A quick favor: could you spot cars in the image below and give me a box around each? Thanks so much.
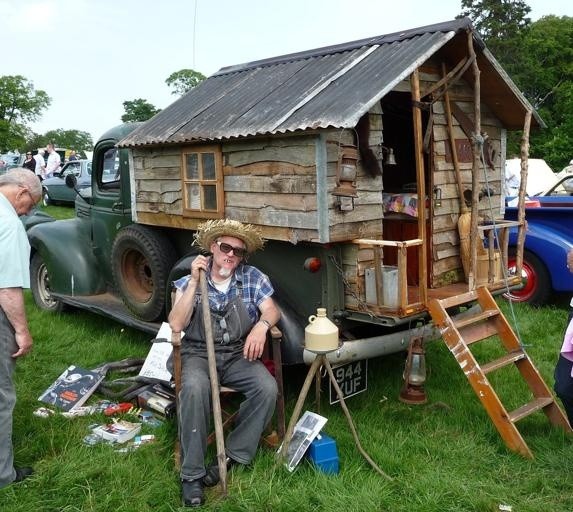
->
[481,156,572,305]
[1,146,121,207]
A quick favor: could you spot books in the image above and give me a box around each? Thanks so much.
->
[32,365,170,454]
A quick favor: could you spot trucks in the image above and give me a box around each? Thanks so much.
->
[22,121,475,406]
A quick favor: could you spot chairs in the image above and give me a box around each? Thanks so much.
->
[170,286,284,469]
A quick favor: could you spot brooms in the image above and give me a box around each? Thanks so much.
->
[442,63,486,282]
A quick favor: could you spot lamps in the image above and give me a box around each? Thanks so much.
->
[397,317,427,404]
[331,128,359,211]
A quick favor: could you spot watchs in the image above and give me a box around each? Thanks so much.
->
[258,319,274,329]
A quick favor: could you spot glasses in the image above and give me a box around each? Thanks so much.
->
[26,154,31,155]
[214,239,246,257]
[18,185,40,210]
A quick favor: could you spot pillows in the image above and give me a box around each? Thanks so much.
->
[383,193,429,217]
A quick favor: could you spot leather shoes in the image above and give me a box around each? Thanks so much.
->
[180,478,204,507]
[12,466,33,483]
[202,450,236,487]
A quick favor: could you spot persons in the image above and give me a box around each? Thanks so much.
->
[554,247,573,431]
[0,143,63,181]
[69,149,77,161]
[167,219,284,508]
[0,166,44,488]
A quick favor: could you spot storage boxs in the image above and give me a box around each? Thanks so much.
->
[307,431,339,475]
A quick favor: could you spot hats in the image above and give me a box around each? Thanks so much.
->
[189,218,265,256]
